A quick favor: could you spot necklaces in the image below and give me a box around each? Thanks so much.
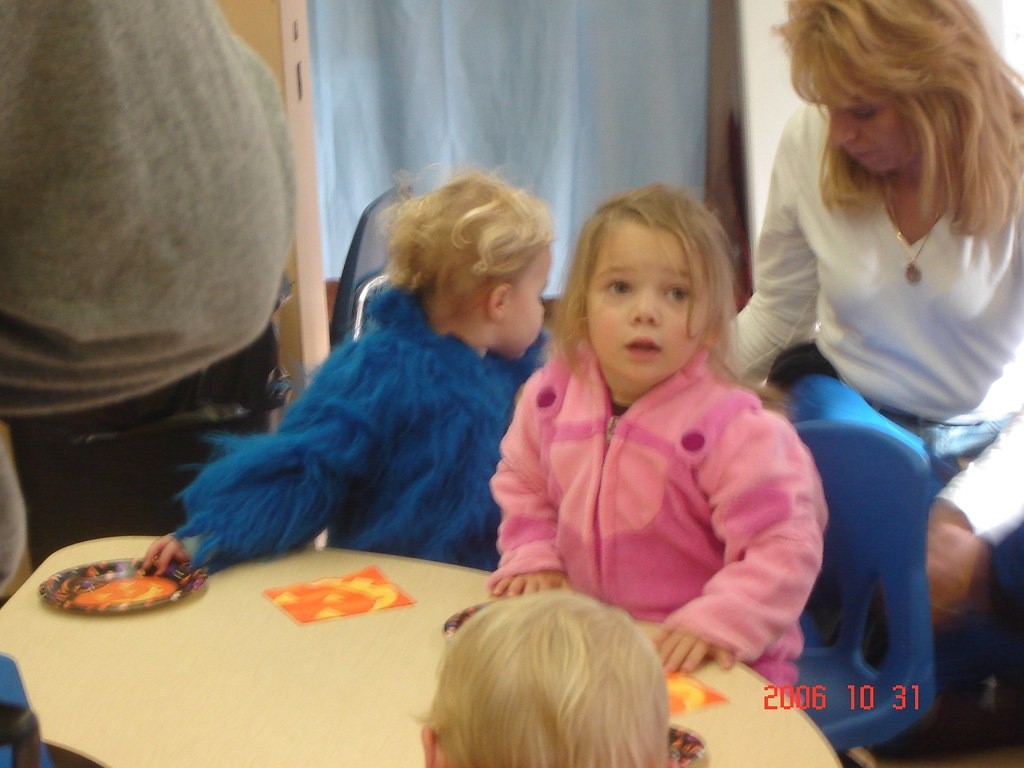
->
[870,174,945,286]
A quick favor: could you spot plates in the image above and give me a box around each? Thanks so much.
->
[38,558,207,612]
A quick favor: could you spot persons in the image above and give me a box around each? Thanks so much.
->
[138,170,555,584]
[712,9,1023,638]
[2,1,298,568]
[485,187,829,689]
[423,591,674,768]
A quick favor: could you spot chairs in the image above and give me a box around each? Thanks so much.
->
[330,186,411,348]
[785,372,937,768]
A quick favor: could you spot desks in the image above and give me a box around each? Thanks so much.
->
[0,534,844,768]
